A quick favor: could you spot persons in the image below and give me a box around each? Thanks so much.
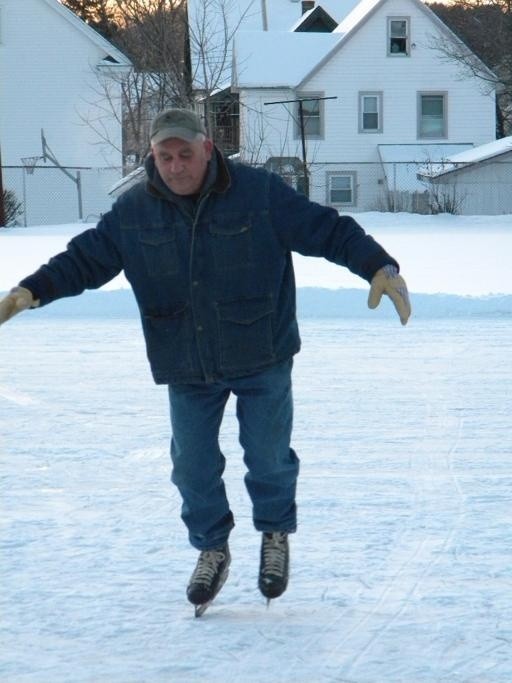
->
[0,108,414,606]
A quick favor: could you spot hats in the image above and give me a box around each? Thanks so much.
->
[148,107,206,147]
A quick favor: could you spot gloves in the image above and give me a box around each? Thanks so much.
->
[366,261,411,328]
[0,285,40,326]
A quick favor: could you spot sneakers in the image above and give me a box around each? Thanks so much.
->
[186,540,232,606]
[257,528,291,599]
